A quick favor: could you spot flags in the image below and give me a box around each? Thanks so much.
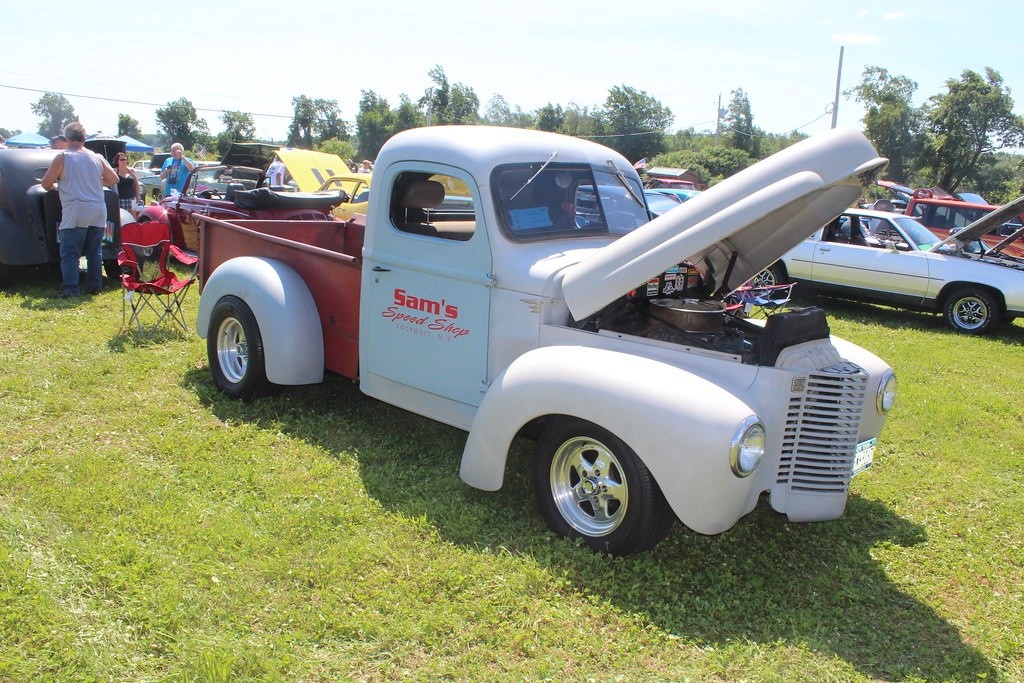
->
[634,158,645,169]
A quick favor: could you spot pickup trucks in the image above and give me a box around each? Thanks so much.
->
[192,118,900,557]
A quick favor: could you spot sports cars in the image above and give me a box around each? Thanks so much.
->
[135,142,348,263]
[840,214,1024,261]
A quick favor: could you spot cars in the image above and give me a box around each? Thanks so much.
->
[270,147,373,223]
[128,147,221,196]
[0,147,146,284]
[570,177,706,228]
[746,196,1024,335]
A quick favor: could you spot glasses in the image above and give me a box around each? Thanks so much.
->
[119,159,127,161]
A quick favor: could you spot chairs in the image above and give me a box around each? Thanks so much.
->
[397,180,445,235]
[735,278,798,318]
[114,222,200,338]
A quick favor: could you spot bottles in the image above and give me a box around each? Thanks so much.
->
[169,188,181,197]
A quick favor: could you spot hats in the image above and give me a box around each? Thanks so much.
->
[51,135,66,142]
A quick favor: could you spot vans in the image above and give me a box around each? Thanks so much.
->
[902,197,1023,258]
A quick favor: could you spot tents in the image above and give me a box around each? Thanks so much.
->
[118,136,155,156]
[85,130,127,167]
[5,132,52,147]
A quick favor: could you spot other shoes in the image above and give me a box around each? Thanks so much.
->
[61,290,80,298]
[86,286,101,295]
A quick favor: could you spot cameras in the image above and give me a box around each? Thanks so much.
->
[167,174,176,184]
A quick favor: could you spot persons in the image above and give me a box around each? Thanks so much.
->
[159,143,195,197]
[42,123,119,297]
[0,135,8,149]
[114,153,139,219]
[347,159,371,173]
[266,155,292,186]
[643,178,649,189]
